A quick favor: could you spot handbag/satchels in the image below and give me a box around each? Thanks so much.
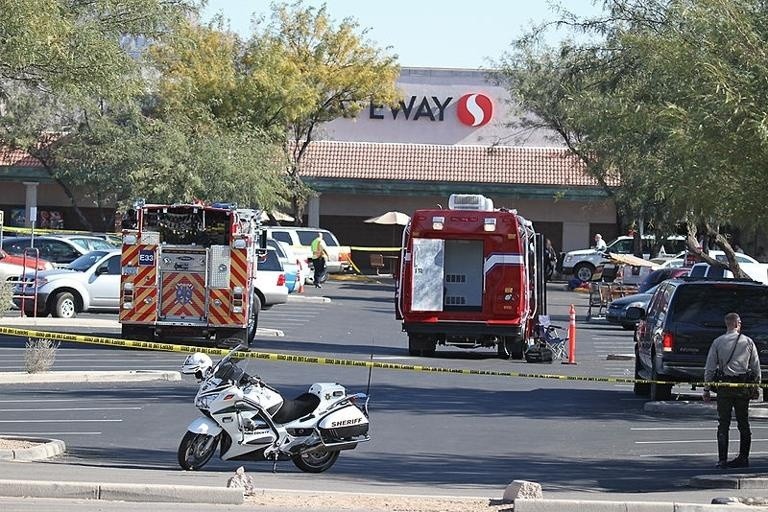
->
[710,368,723,392]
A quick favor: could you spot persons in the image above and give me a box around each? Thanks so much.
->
[595,234,606,250]
[544,238,558,282]
[703,313,762,468]
[311,233,330,288]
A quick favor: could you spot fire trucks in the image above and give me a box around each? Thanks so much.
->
[393,191,556,362]
[117,197,271,350]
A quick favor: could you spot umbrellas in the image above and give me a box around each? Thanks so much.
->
[363,211,412,273]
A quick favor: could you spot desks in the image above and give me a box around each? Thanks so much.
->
[383,256,399,273]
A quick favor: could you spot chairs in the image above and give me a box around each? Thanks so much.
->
[369,254,385,274]
[586,282,637,322]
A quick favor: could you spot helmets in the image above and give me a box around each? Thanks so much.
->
[181,352,212,380]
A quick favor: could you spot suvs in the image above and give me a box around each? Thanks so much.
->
[633,278,768,402]
[562,233,691,284]
[13,249,122,318]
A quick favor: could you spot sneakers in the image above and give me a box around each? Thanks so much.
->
[716,457,748,468]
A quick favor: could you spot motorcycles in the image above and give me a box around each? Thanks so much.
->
[173,339,377,474]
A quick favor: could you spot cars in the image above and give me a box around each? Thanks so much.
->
[602,248,768,328]
[0,223,122,308]
[253,220,351,314]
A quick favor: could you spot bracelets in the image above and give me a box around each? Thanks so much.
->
[703,388,710,392]
